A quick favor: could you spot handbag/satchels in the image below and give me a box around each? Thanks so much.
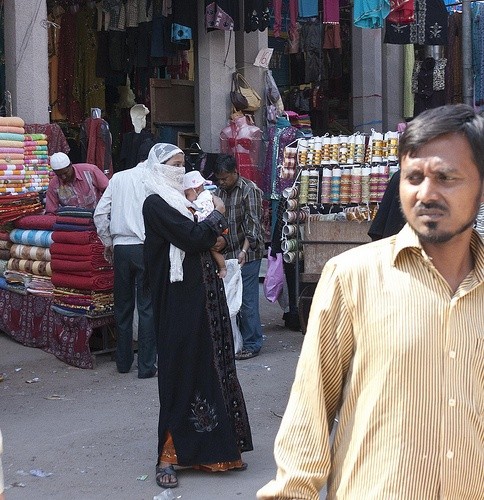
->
[263,246,285,303]
[230,72,261,111]
[264,71,279,103]
[277,265,290,313]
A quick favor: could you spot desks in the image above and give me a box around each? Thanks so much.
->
[0,277,139,369]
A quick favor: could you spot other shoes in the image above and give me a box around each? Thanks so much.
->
[235,350,258,360]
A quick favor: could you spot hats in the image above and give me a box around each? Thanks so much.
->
[50,152,71,170]
[183,170,212,191]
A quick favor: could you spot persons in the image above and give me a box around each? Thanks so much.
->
[92,138,158,379]
[183,169,228,278]
[257,103,484,500]
[260,116,305,202]
[210,153,265,359]
[117,104,155,173]
[221,115,264,188]
[83,109,113,181]
[141,143,254,488]
[44,152,110,214]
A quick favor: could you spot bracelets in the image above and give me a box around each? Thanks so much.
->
[242,249,246,253]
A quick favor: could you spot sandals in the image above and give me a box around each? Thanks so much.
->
[229,462,248,471]
[156,464,178,488]
